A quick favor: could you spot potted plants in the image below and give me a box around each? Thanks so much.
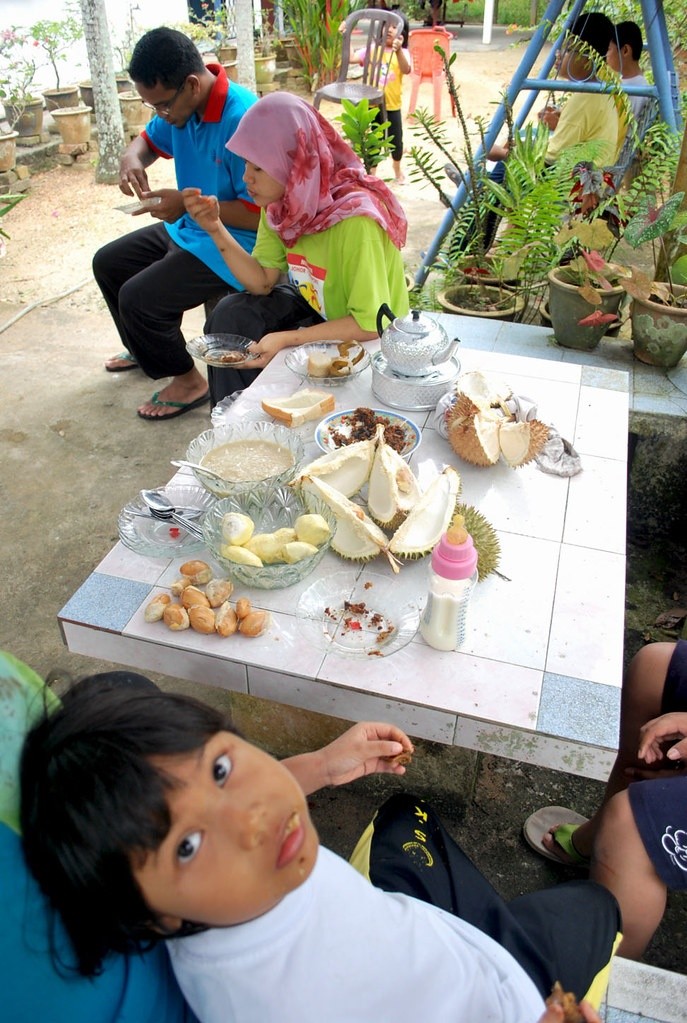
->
[408,19,687,367]
[0,0,286,173]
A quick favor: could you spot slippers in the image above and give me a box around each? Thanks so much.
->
[138,389,210,420]
[106,351,139,372]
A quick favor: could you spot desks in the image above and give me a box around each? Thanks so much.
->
[58,326,629,781]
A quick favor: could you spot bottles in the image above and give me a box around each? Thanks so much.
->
[418,513,479,652]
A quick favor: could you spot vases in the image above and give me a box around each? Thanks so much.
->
[280,37,303,68]
[78,80,95,114]
[50,105,93,143]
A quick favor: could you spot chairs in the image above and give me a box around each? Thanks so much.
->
[314,9,404,153]
[407,26,456,126]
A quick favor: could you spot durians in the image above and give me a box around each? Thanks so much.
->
[220,510,329,567]
[447,392,551,465]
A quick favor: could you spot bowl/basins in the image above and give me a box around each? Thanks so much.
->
[284,339,372,386]
[201,486,337,591]
[314,409,422,459]
[186,420,305,499]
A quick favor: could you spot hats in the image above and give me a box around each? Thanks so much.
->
[570,12,615,62]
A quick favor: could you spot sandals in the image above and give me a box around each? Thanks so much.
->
[521,806,596,871]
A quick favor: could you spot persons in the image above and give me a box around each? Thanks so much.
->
[18,687,624,1023]
[523,639,687,958]
[338,10,413,185]
[445,21,649,189]
[181,91,410,414]
[91,26,259,420]
[419,13,630,269]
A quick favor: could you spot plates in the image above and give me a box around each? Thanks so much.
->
[120,540,206,558]
[117,483,219,550]
[297,569,422,662]
[117,534,206,554]
[186,333,259,368]
[208,382,345,448]
[112,195,162,214]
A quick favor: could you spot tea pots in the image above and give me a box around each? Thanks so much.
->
[376,302,461,377]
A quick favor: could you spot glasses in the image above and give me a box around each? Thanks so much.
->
[141,72,197,116]
[555,47,591,58]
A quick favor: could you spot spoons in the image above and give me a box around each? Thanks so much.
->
[138,488,204,542]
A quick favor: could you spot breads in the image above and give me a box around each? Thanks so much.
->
[261,387,334,426]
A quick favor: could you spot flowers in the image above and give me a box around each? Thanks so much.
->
[0,25,50,101]
